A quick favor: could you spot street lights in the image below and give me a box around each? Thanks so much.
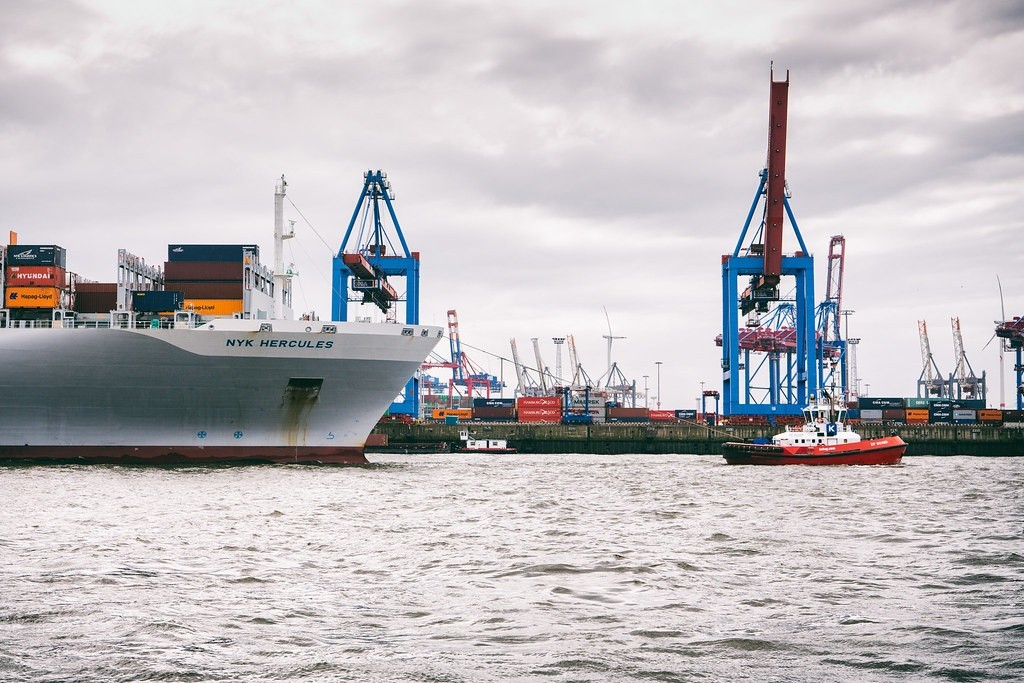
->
[643,375,649,407]
[696,397,701,413]
[700,381,705,391]
[655,362,663,410]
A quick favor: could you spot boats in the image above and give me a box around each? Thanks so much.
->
[460,439,517,454]
[722,385,910,466]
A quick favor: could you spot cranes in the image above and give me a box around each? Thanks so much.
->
[918,315,988,402]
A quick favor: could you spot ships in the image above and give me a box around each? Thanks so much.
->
[0,244,444,471]
